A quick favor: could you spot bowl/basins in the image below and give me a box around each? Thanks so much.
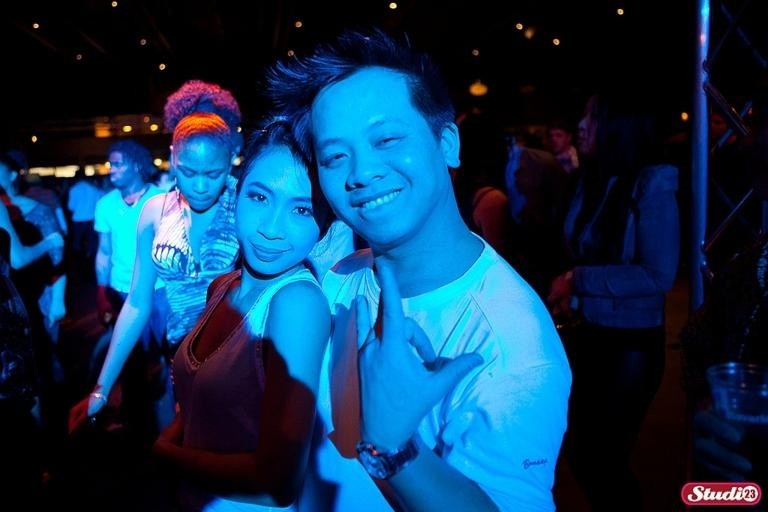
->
[709,360,768,427]
